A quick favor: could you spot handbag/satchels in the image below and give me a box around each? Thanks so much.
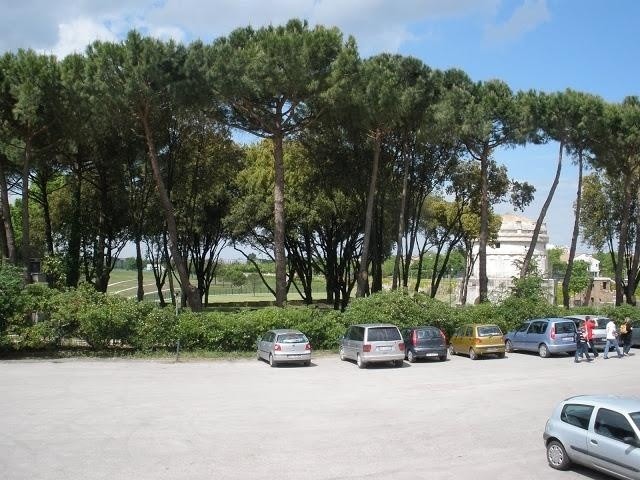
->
[620,325,628,334]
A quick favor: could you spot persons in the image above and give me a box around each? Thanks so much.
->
[603,317,624,359]
[575,316,600,363]
[622,316,632,355]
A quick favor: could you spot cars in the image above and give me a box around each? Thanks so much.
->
[617,320,640,348]
[564,314,616,350]
[448,323,506,360]
[504,318,578,358]
[542,394,640,480]
[258,328,311,366]
[400,326,449,361]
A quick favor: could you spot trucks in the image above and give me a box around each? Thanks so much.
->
[146,264,166,271]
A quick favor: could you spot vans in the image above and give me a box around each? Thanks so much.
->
[337,323,406,368]
